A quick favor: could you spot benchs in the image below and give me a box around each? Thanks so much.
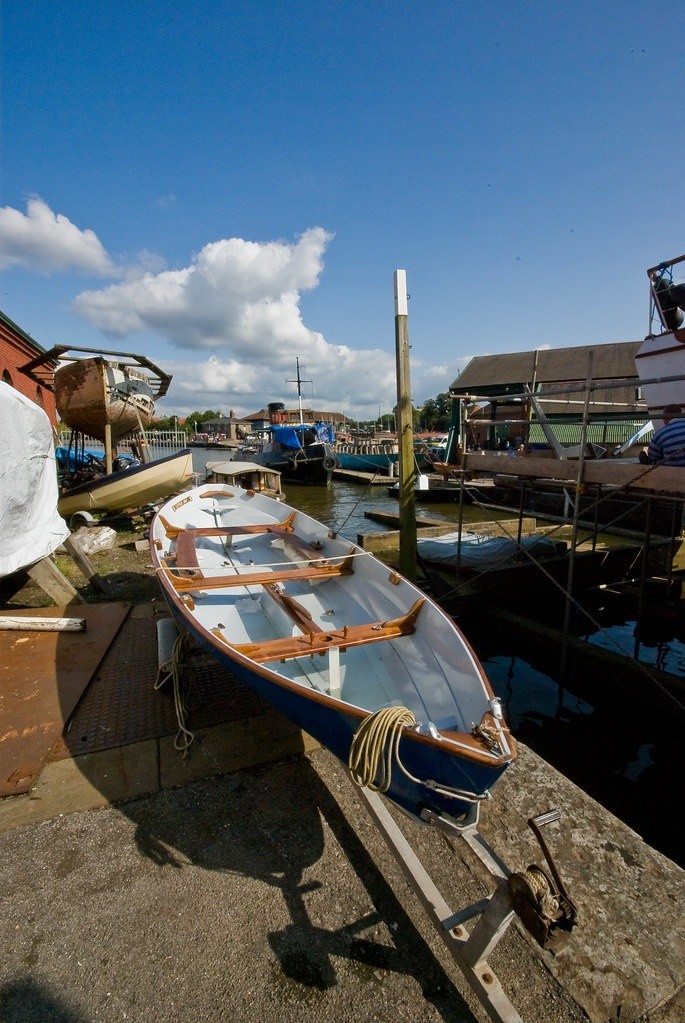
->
[162,545,354,593]
[177,529,203,579]
[268,528,335,567]
[212,597,425,662]
[159,510,296,538]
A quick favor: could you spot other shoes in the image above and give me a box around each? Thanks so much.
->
[639,451,648,465]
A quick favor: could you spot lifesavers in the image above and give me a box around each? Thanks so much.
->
[287,460,297,472]
[322,456,337,472]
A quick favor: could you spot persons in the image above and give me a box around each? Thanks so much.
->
[639,404,685,466]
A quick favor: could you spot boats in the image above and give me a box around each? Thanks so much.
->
[57,447,194,519]
[334,438,446,473]
[230,357,338,485]
[201,461,287,504]
[386,482,481,502]
[150,481,519,835]
[52,356,157,445]
